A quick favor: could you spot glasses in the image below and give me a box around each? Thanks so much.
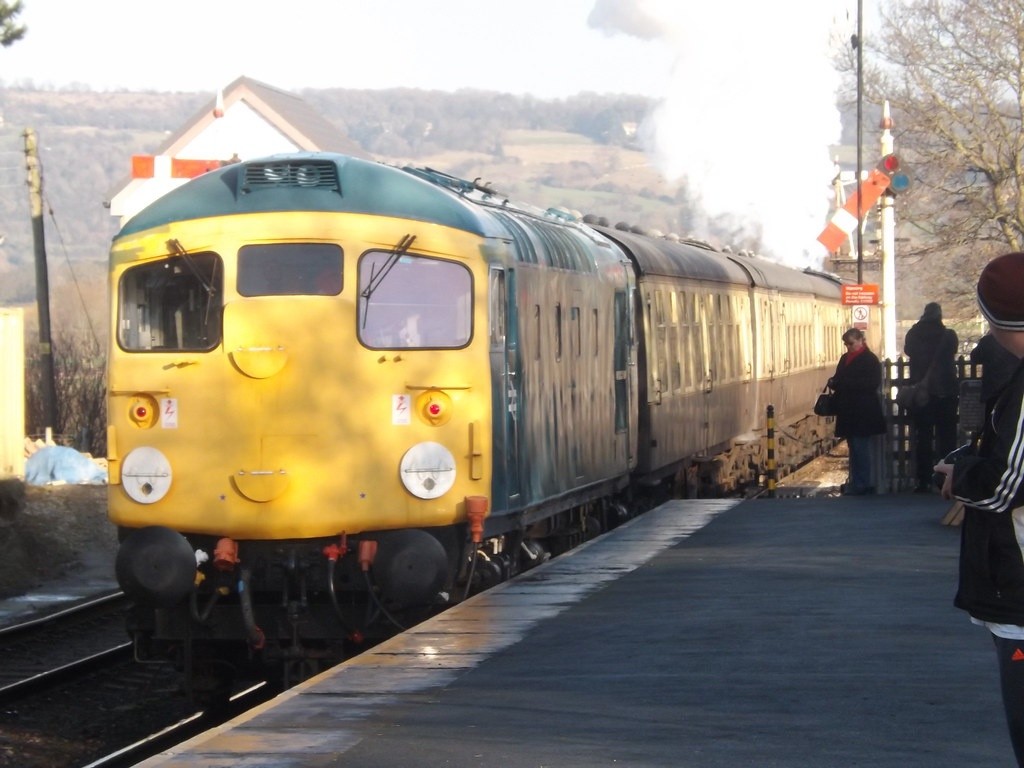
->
[844,342,854,345]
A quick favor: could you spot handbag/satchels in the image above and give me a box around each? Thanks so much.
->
[814,385,837,416]
[896,384,928,410]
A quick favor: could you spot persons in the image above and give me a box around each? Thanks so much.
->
[970,329,1020,423]
[904,302,958,492]
[826,328,882,497]
[932,255,1024,767]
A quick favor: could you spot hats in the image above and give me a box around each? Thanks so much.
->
[977,253,1024,332]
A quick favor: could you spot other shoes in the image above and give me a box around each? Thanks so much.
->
[848,489,877,505]
[913,484,931,496]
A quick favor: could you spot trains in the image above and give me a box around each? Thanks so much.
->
[95,152,884,670]
